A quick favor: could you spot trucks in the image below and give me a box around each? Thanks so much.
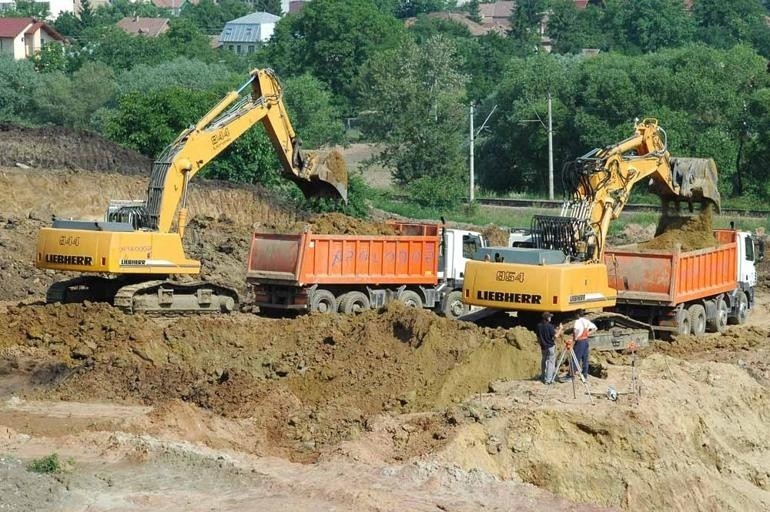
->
[600,220,765,344]
[247,214,488,323]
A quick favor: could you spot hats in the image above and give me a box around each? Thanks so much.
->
[543,312,553,318]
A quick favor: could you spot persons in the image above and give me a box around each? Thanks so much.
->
[536,311,563,385]
[561,309,599,383]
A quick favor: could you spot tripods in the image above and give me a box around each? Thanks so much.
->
[542,345,595,405]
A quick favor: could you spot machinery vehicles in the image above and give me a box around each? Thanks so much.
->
[459,116,720,353]
[39,64,350,313]
